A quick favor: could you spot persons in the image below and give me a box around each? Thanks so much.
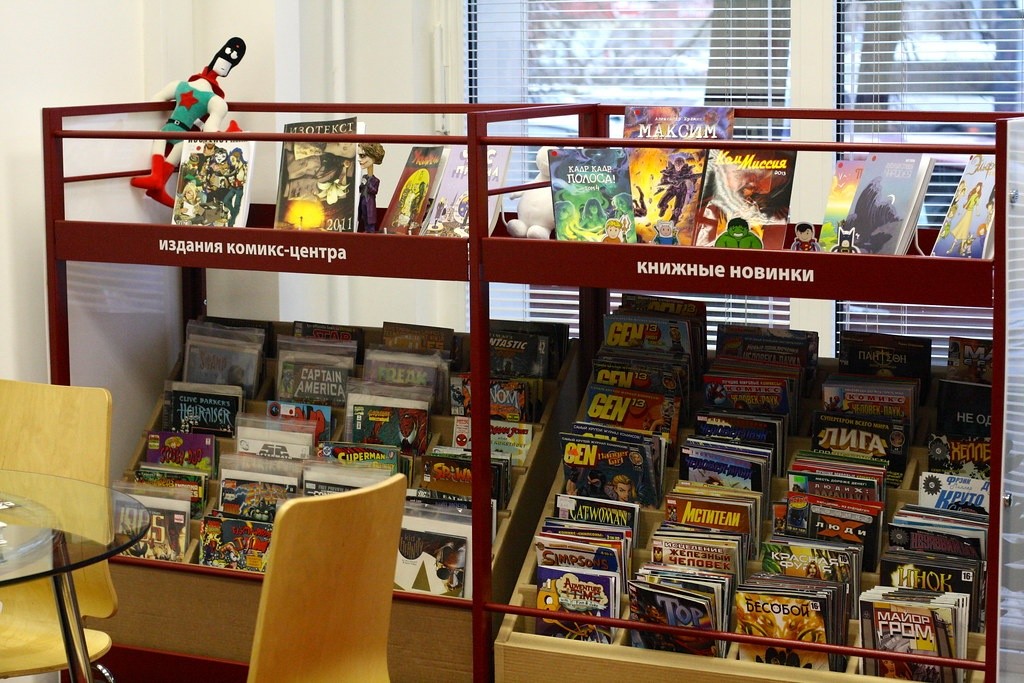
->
[356,143,385,233]
[130,36,246,208]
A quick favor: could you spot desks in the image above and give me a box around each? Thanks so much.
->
[0,468,150,683]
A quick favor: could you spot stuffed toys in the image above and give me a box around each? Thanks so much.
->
[501,146,563,240]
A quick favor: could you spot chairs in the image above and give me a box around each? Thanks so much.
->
[248,475,407,683]
[0,377,118,683]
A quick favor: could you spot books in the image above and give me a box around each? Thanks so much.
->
[273,116,366,235]
[548,106,800,249]
[819,152,936,255]
[379,145,515,239]
[172,140,256,229]
[931,155,996,259]
[110,291,993,683]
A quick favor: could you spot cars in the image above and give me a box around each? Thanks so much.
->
[526,119,623,182]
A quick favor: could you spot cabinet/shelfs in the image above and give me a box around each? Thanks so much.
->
[43,101,1024,681]
